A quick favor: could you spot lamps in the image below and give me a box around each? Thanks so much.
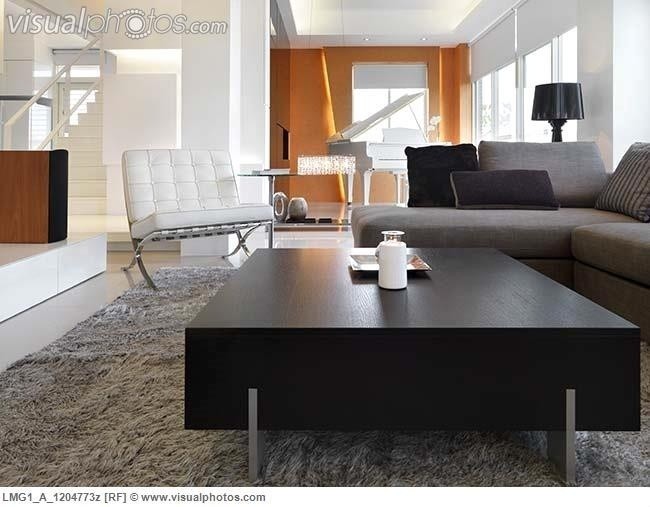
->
[531,83,584,142]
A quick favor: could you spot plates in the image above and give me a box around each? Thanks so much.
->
[348,254,433,274]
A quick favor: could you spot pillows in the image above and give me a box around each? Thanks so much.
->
[450,169,561,209]
[405,144,478,208]
[594,142,650,222]
[478,141,608,208]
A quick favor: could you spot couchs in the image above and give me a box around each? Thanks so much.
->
[351,203,643,292]
[571,222,650,349]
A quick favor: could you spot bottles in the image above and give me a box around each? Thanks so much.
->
[377,230,408,291]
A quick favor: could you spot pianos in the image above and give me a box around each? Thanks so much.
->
[325,93,453,208]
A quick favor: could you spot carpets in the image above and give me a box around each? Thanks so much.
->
[0,267,650,487]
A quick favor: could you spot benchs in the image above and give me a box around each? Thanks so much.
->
[121,150,274,289]
